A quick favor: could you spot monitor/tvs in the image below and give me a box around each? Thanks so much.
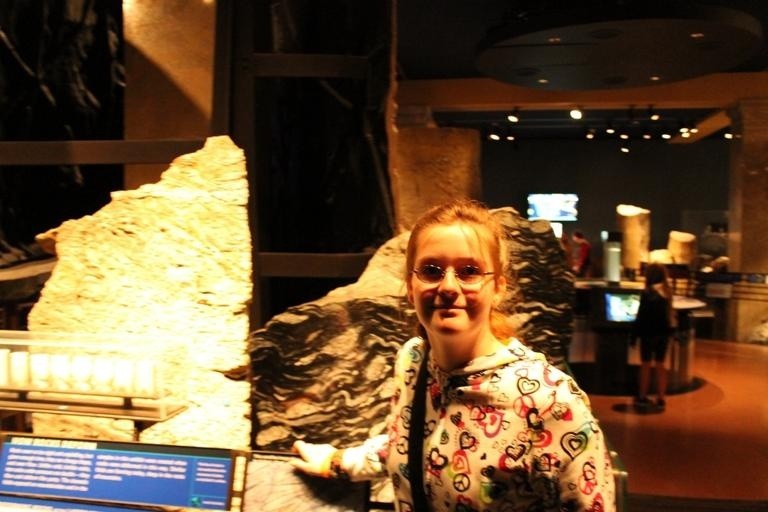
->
[525,192,580,223]
[601,290,642,323]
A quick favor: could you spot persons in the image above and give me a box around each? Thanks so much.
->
[572,229,597,279]
[628,260,677,412]
[560,233,575,267]
[288,199,618,512]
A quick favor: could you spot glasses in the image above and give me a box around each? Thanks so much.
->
[411,264,499,284]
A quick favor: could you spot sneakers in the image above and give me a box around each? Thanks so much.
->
[635,398,665,409]
